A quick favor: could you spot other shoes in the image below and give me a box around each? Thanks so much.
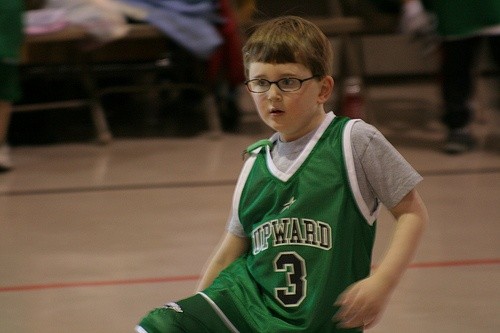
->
[440,130,476,152]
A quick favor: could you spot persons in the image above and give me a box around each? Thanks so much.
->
[401,0,500,154]
[135,21,429,333]
[1,0,25,173]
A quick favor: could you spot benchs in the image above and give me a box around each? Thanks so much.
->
[1,17,224,143]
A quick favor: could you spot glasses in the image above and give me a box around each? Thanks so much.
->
[244,74,318,94]
